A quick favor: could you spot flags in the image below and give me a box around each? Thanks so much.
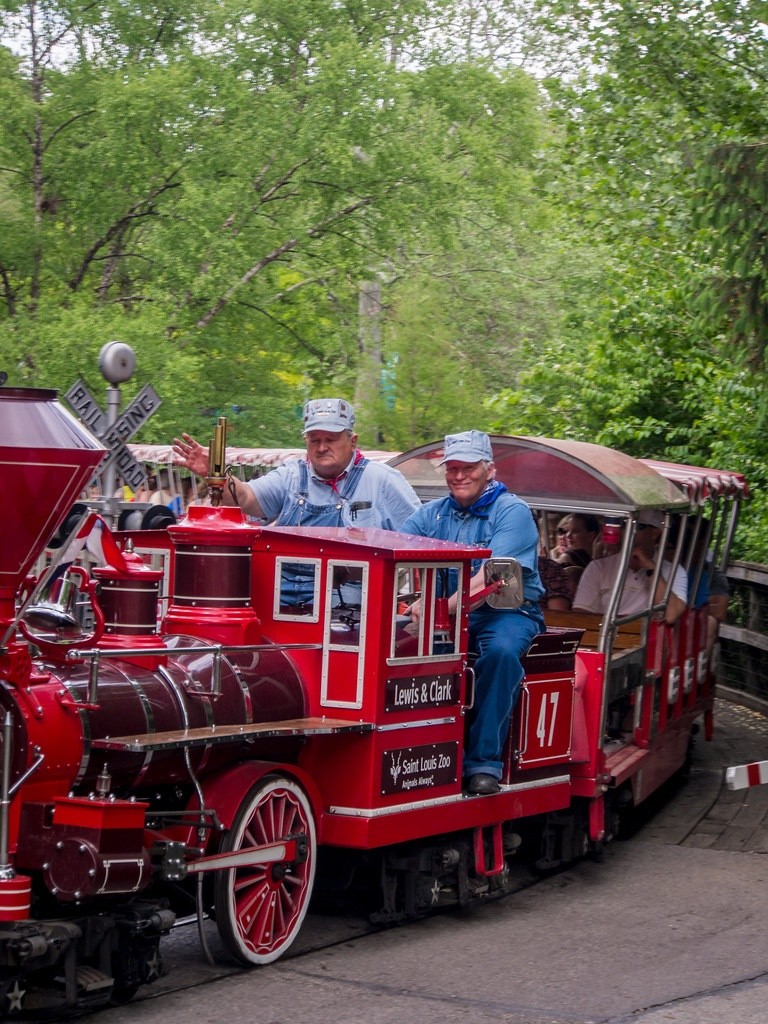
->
[59,513,131,576]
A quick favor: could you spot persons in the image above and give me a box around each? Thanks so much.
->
[171,398,424,613]
[538,512,729,741]
[401,429,547,796]
[89,467,207,518]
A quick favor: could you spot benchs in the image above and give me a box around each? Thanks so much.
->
[527,626,586,674]
[545,612,647,740]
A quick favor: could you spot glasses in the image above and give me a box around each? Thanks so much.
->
[558,527,568,535]
[622,522,656,530]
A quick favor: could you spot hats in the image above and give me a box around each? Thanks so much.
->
[300,399,355,435]
[439,430,492,466]
[639,510,665,528]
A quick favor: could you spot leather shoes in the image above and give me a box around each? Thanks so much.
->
[468,773,499,793]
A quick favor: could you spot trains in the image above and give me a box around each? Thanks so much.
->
[0,342,750,1024]
